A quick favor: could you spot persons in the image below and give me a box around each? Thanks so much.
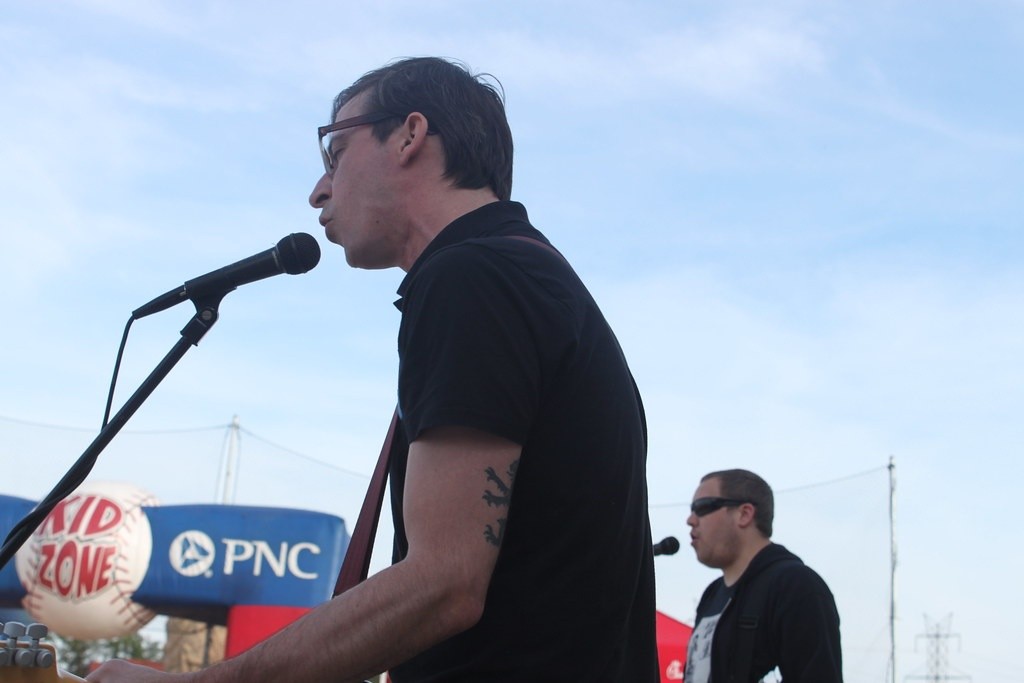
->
[77,54,659,682]
[682,468,842,683]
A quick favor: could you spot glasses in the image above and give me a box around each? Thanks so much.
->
[689,495,759,515]
[318,110,440,178]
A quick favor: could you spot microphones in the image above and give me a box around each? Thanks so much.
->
[653,537,680,555]
[133,233,321,319]
